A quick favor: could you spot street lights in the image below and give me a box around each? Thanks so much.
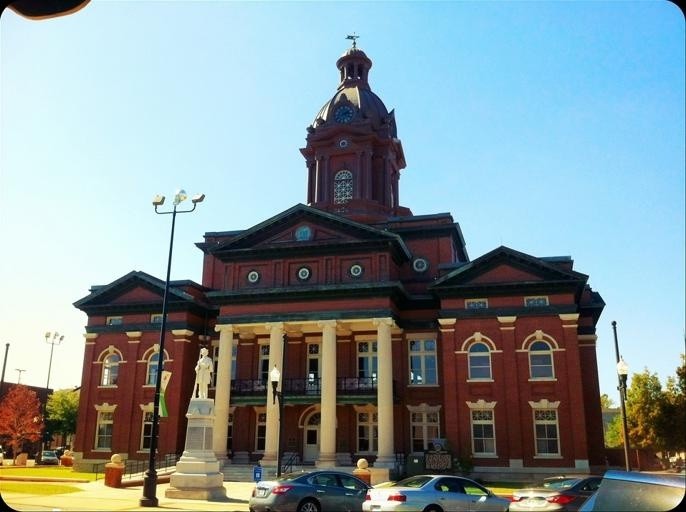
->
[35,332,63,466]
[136,188,206,508]
[269,365,282,477]
[610,319,632,470]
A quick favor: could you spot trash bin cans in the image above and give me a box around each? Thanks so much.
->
[254,467,261,481]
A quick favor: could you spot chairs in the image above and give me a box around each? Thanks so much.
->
[326,479,339,486]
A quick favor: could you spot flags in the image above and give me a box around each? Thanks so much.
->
[157,370,172,418]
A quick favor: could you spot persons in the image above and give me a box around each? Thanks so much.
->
[194,347,213,399]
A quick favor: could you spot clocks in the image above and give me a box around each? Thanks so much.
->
[332,101,356,124]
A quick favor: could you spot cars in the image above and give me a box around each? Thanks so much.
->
[0,445,6,465]
[508,476,603,512]
[362,475,512,512]
[30,446,70,466]
[249,469,372,512]
